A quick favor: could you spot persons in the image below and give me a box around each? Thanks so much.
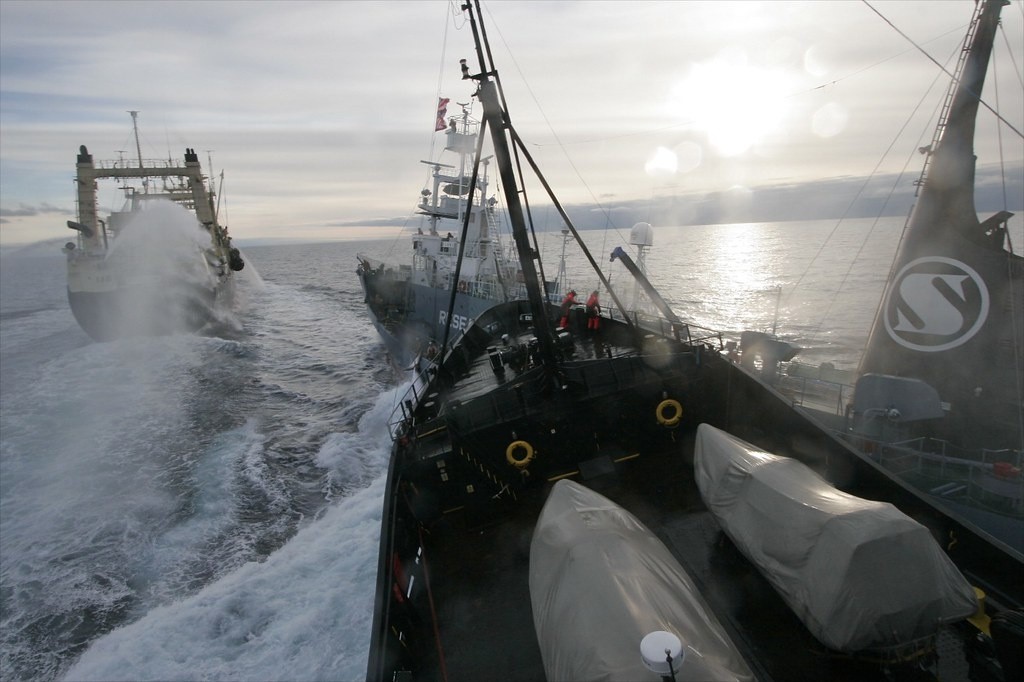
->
[586,290,601,332]
[559,289,584,330]
[418,227,422,236]
[946,528,968,577]
[223,226,228,239]
[447,232,454,252]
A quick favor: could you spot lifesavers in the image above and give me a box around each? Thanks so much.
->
[656,399,683,426]
[506,439,534,468]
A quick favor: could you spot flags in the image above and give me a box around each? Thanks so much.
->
[435,97,450,132]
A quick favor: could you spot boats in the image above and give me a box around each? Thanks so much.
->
[56,109,244,341]
[350,0,1024,682]
